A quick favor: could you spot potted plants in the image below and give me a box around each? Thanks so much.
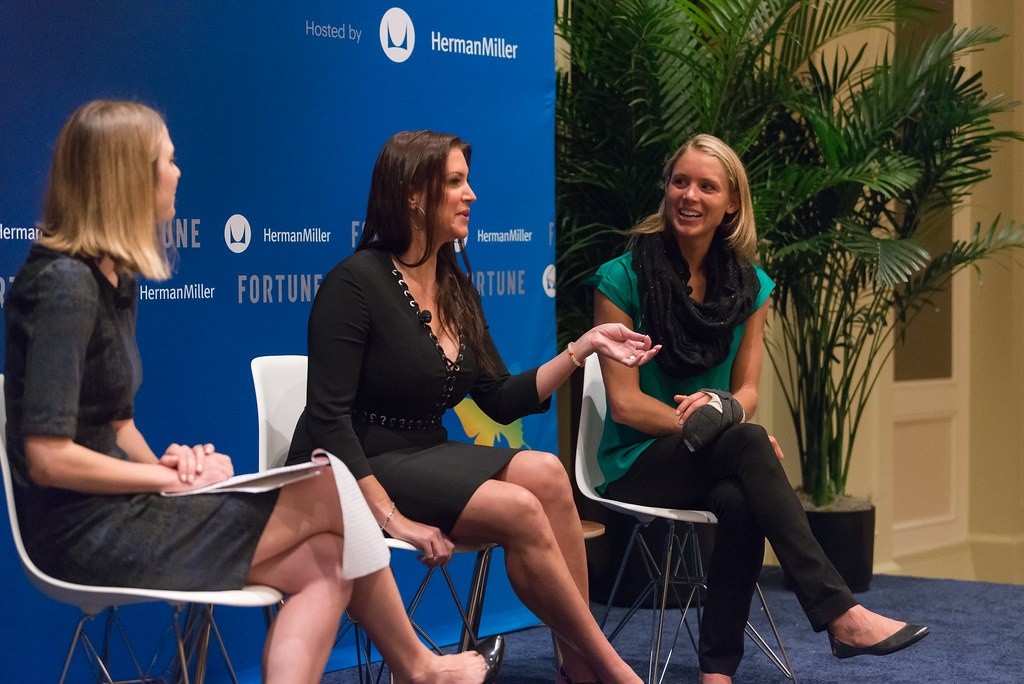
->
[757,30,1024,592]
[553,0,942,609]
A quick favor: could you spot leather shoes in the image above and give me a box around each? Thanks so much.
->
[827,621,930,659]
[467,635,504,684]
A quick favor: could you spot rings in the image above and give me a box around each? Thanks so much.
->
[630,356,636,360]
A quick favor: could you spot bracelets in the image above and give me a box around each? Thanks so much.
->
[380,502,395,530]
[567,342,586,368]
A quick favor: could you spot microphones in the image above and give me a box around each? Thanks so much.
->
[115,293,132,310]
[420,310,431,323]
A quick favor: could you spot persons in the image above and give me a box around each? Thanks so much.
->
[0,101,504,684]
[594,134,931,684]
[285,130,644,684]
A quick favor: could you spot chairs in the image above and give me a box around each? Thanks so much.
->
[249,356,494,683]
[0,373,283,684]
[573,352,796,684]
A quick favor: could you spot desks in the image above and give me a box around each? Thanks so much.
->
[459,521,608,669]
